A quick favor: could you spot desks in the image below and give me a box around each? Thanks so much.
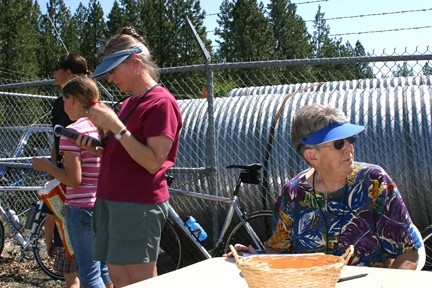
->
[122,253,432,288]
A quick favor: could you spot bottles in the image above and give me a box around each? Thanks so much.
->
[5,206,23,228]
[184,215,208,242]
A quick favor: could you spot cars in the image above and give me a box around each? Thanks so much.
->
[0,126,57,217]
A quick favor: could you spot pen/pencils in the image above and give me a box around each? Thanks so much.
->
[224,248,262,252]
[337,273,368,283]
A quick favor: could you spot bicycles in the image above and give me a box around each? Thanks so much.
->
[0,204,64,281]
[156,163,296,276]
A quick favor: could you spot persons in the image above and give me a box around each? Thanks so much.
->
[31,52,114,288]
[86,26,183,288]
[224,104,427,271]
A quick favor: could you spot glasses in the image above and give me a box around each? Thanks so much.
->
[55,64,65,71]
[320,136,356,150]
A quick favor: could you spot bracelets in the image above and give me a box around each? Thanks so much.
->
[115,126,127,140]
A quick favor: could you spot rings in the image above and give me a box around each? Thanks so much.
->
[92,114,96,119]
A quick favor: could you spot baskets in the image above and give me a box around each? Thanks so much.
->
[229,244,355,288]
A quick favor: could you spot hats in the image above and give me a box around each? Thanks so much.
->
[89,48,143,79]
[299,120,365,147]
